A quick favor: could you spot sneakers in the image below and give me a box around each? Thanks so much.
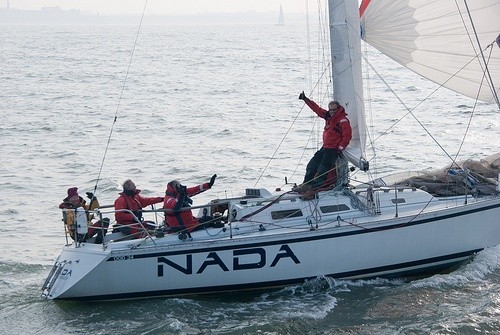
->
[292,183,309,190]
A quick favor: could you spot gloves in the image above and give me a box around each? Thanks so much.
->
[298,91,304,100]
[134,214,140,221]
[336,148,341,154]
[209,174,217,188]
[86,192,93,199]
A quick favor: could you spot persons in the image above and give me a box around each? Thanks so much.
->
[113,180,164,236]
[162,173,218,234]
[59,186,110,245]
[292,90,353,199]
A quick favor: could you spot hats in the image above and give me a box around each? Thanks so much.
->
[67,187,78,198]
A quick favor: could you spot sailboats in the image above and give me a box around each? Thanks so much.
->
[40,1,500,299]
